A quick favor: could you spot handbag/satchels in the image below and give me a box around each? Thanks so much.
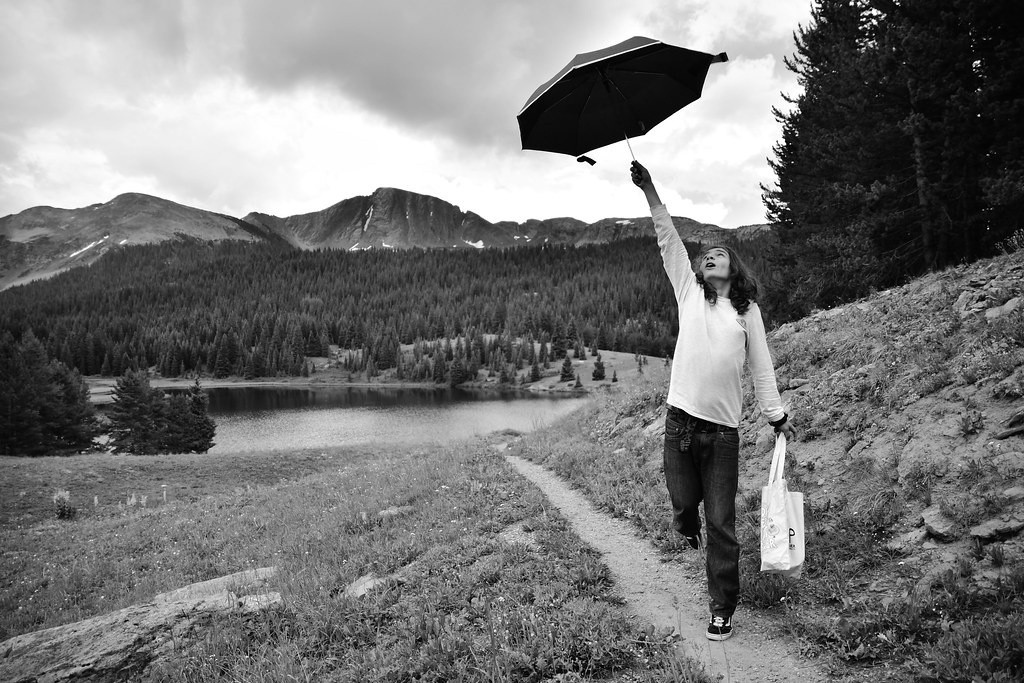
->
[760,432,804,579]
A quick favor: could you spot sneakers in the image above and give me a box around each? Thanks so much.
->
[706,614,732,641]
[685,532,702,550]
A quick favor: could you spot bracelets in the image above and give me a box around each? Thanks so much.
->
[768,413,789,429]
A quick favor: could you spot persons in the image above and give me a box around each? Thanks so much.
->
[629,160,799,641]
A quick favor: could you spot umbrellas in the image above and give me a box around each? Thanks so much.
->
[516,35,729,179]
